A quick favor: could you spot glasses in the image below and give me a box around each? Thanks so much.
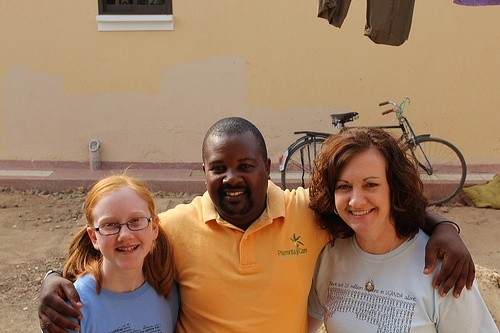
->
[94,216,153,237]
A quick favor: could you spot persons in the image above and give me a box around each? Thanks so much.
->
[37,117,476,333]
[42,173,179,332]
[307,127,499,332]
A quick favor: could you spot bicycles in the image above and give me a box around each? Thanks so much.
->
[278,101,467,205]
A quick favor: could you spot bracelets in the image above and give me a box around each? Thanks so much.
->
[428,219,460,236]
[41,269,64,286]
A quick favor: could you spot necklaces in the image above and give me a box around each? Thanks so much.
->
[355,238,398,291]
